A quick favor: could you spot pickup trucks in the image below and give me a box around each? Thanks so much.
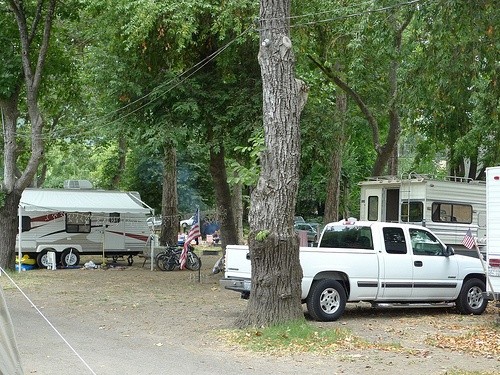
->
[220,221,488,321]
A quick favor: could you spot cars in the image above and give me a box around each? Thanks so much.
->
[180,216,195,228]
[294,223,317,242]
[147,217,162,228]
[294,216,305,224]
[178,232,186,246]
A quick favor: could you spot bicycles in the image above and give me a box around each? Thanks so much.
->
[155,241,202,271]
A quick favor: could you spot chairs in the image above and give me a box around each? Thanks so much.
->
[340,235,371,250]
